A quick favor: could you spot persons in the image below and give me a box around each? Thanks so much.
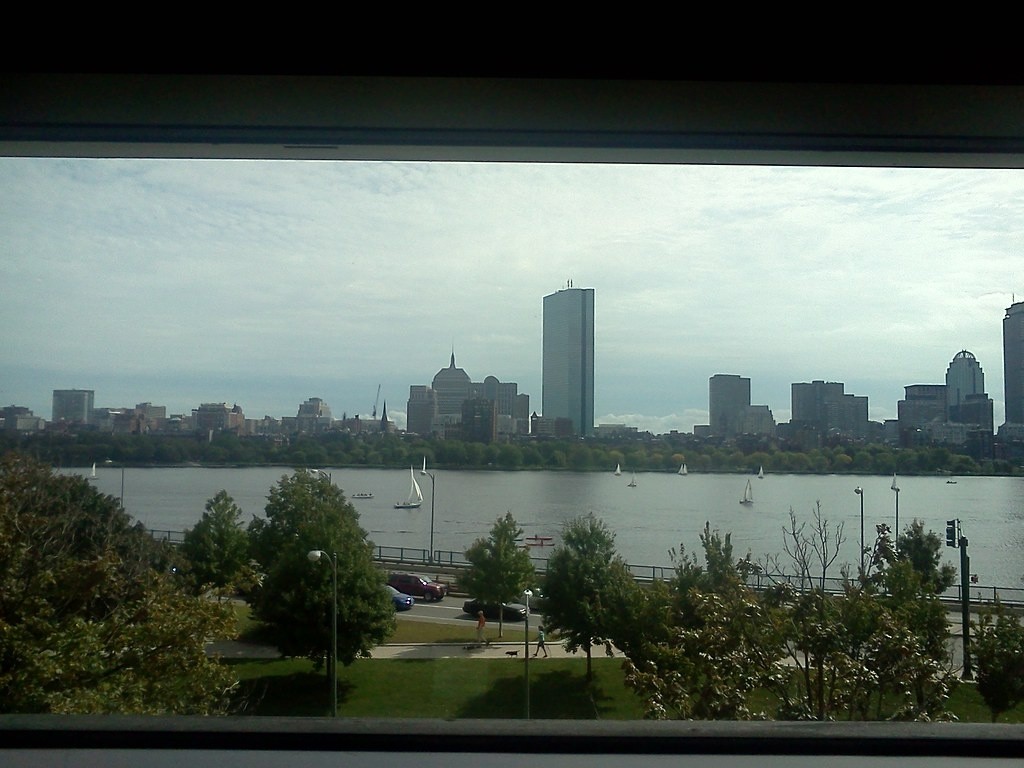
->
[533,625,548,657]
[475,611,489,647]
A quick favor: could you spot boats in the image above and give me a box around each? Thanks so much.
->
[351,493,375,498]
[946,480,957,484]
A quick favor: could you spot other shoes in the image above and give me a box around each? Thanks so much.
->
[544,655,547,657]
[533,654,537,656]
[476,646,481,648]
[486,642,489,646]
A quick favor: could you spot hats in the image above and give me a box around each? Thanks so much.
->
[478,611,483,614]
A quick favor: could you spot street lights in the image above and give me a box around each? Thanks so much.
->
[105,459,124,508]
[854,486,865,573]
[891,484,901,552]
[524,588,533,717]
[307,548,338,717]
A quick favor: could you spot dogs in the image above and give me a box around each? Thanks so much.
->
[505,650,520,658]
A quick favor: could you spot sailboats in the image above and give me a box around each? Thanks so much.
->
[757,465,764,479]
[393,465,424,509]
[420,456,427,475]
[740,479,753,504]
[677,463,688,475]
[614,463,622,476]
[627,470,637,487]
[89,462,99,480]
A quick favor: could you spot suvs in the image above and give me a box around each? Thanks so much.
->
[386,572,448,603]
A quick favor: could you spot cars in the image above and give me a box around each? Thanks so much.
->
[463,596,530,622]
[381,583,414,612]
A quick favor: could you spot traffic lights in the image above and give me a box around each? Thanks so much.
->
[945,518,958,548]
